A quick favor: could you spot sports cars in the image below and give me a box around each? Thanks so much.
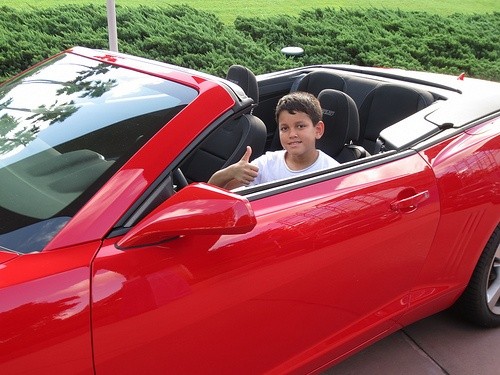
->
[0,46,500,375]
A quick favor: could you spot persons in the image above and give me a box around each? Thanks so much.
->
[206,92,341,191]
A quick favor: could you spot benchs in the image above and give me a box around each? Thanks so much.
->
[269,70,434,164]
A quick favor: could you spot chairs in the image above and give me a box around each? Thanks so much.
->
[314,88,372,164]
[182,65,267,184]
